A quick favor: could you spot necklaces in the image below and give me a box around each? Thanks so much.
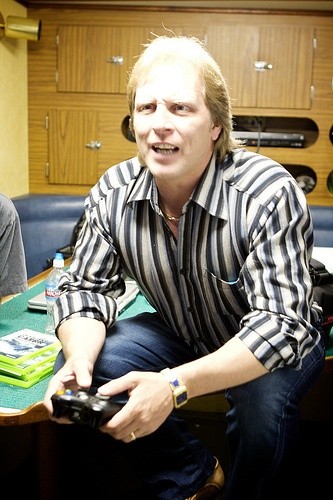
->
[159,201,180,222]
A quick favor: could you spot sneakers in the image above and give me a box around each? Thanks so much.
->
[178,455,227,500]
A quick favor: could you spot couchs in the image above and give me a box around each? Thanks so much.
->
[8,190,333,288]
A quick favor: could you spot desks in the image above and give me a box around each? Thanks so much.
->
[0,256,159,428]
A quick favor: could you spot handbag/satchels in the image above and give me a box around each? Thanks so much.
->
[308,258,333,348]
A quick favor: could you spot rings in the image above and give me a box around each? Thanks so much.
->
[130,432,136,441]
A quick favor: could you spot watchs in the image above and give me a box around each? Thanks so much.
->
[160,367,188,409]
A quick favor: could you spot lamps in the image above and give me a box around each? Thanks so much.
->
[0,11,42,42]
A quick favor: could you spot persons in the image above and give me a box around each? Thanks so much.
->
[42,35,325,500]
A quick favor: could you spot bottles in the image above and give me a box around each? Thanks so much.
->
[45,253,65,334]
